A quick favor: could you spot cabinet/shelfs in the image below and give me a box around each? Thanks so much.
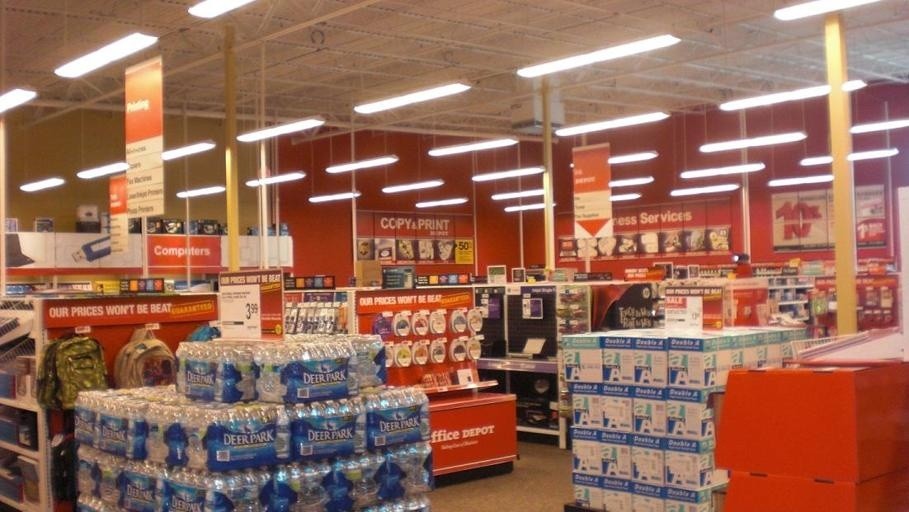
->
[282,287,356,339]
[813,273,901,332]
[357,283,516,488]
[474,285,593,450]
[769,275,815,332]
[654,277,768,327]
[1,295,61,511]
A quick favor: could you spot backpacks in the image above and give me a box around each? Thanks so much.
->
[113,328,176,389]
[34,332,108,412]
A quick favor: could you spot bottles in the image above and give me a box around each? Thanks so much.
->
[72,331,433,512]
